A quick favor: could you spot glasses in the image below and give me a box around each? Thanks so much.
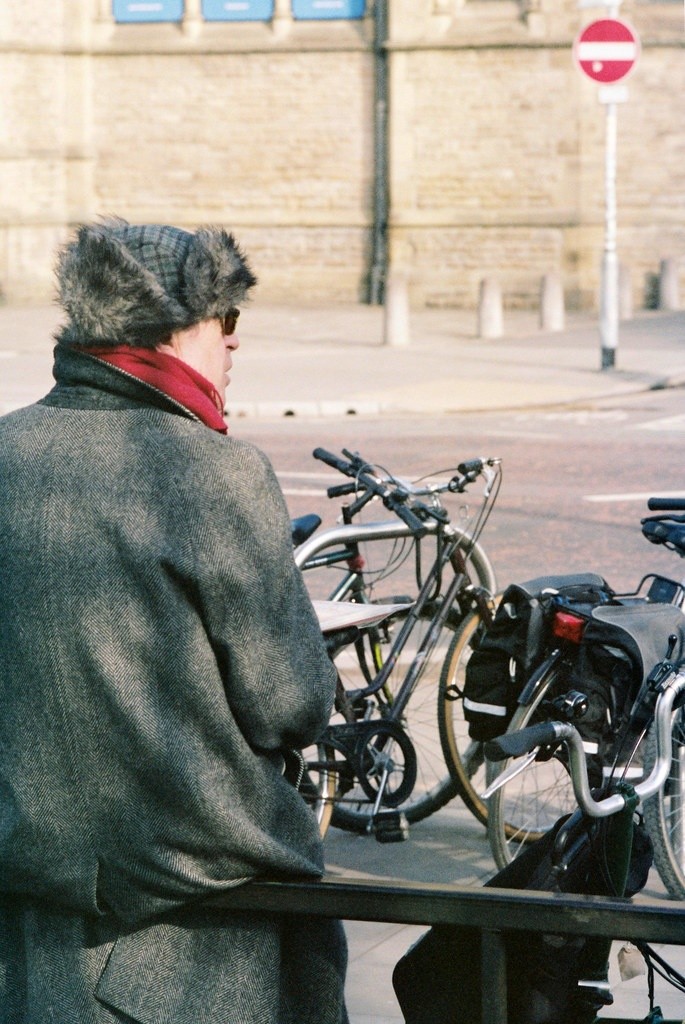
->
[212,308,240,336]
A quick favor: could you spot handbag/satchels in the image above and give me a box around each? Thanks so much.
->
[568,601,684,786]
[390,789,654,1024]
[462,570,610,741]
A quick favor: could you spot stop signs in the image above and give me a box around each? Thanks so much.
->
[575,19,641,83]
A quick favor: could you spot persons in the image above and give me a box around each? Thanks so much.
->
[0,218,350,1024]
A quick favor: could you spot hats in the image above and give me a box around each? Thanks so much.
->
[54,216,255,345]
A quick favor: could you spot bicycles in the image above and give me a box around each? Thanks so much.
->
[485,632,684,1024]
[285,446,685,900]
